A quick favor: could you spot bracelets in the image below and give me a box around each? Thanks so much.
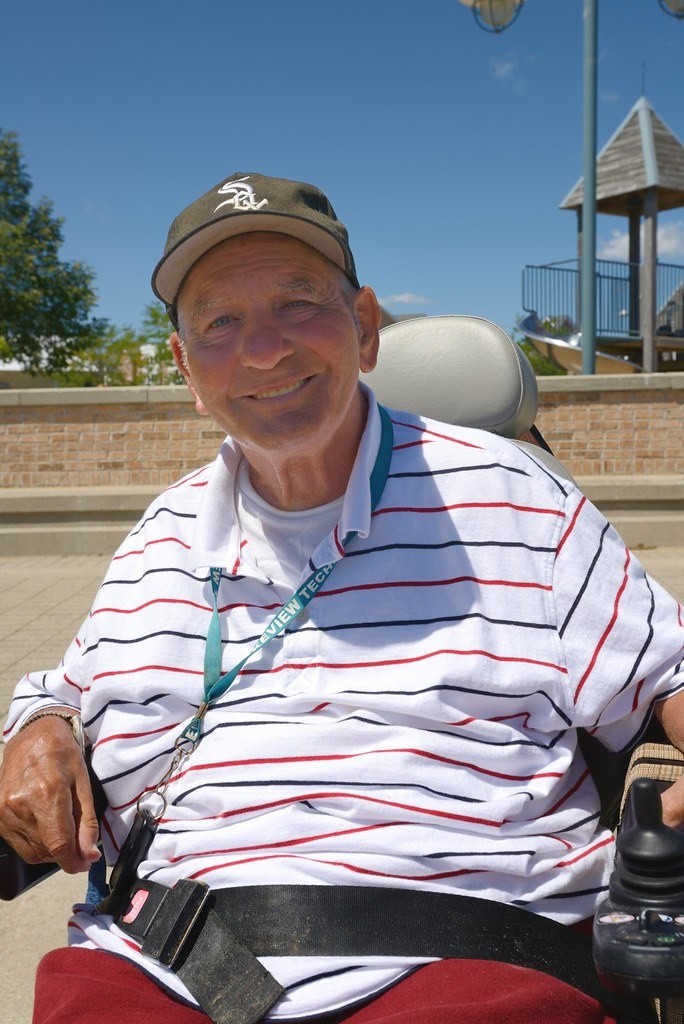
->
[19,709,86,761]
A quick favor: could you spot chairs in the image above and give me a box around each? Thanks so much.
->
[0,314,684,1024]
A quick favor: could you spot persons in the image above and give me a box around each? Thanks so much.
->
[0,172,684,1024]
[658,301,678,360]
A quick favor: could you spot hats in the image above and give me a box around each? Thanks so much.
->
[152,172,360,333]
